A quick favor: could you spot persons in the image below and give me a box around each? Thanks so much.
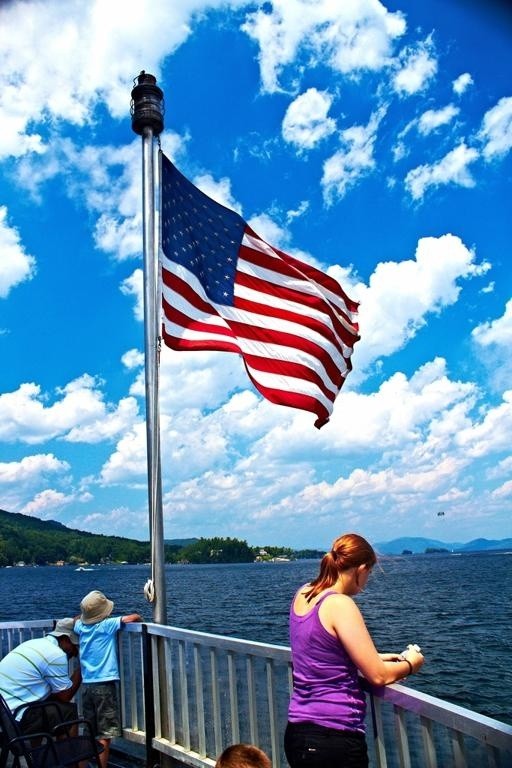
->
[0,618,82,752]
[283,533,425,768]
[72,589,143,767]
[214,744,272,768]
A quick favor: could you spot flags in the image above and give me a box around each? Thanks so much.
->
[158,154,363,433]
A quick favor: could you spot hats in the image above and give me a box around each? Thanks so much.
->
[45,618,80,646]
[79,590,114,626]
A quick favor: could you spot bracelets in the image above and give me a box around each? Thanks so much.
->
[403,658,413,678]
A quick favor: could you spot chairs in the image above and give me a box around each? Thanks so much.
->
[1,694,107,767]
